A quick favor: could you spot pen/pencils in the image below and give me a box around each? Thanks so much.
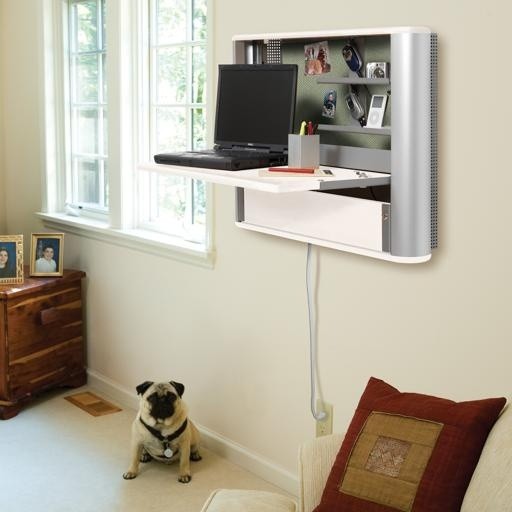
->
[299,120,319,135]
[269,167,315,173]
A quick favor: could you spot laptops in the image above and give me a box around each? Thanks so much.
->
[154,63,299,171]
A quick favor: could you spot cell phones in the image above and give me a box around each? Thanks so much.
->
[344,93,365,120]
[341,43,363,73]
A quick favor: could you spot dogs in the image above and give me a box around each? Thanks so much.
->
[121,380,201,484]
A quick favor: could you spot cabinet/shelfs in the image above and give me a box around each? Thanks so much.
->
[136,23,438,264]
[0,264,88,422]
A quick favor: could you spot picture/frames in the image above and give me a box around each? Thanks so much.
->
[0,231,65,286]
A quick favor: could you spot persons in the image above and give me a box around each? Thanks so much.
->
[36,245,57,272]
[323,92,334,115]
[372,64,383,78]
[0,248,15,278]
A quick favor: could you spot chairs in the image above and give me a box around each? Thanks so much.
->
[200,403,511,512]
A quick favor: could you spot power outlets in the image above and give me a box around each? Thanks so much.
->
[315,403,334,438]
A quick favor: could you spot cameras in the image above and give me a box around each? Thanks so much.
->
[365,62,387,78]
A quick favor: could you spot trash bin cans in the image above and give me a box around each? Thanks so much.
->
[200,488,299,512]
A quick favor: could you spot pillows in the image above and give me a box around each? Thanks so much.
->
[308,376,508,511]
